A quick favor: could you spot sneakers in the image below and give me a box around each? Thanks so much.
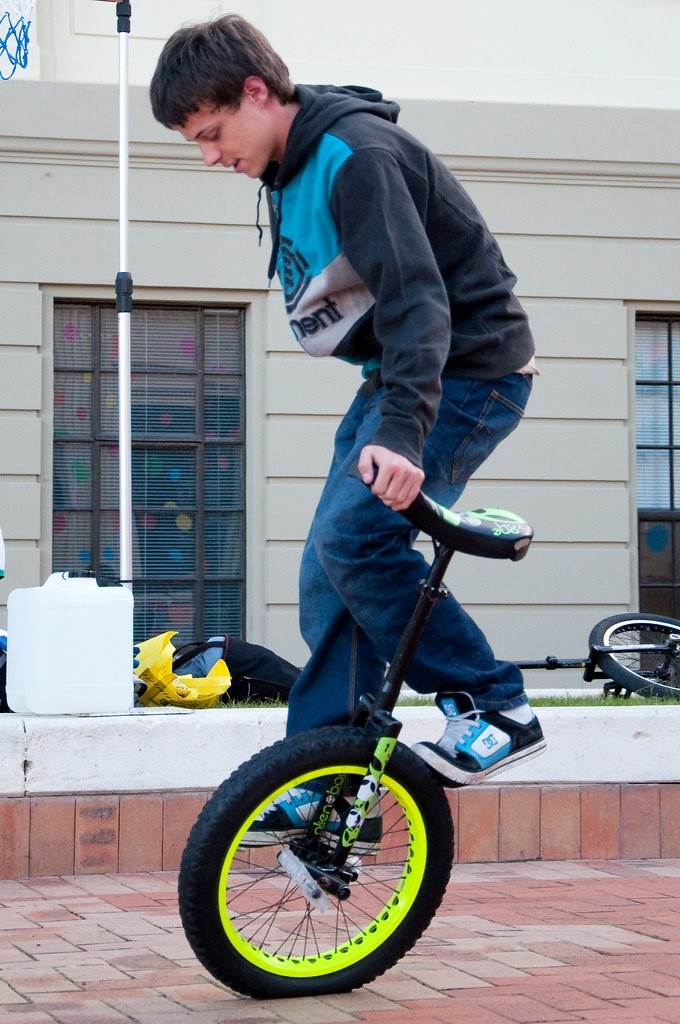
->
[237,786,383,855]
[411,691,548,787]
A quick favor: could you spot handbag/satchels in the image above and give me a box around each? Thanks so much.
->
[172,632,303,703]
[134,631,232,709]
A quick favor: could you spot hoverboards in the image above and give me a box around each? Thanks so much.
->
[175,463,531,999]
[502,613,680,698]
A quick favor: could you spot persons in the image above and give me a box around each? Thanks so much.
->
[146,12,549,851]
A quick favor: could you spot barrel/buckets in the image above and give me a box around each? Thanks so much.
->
[6,570,135,715]
